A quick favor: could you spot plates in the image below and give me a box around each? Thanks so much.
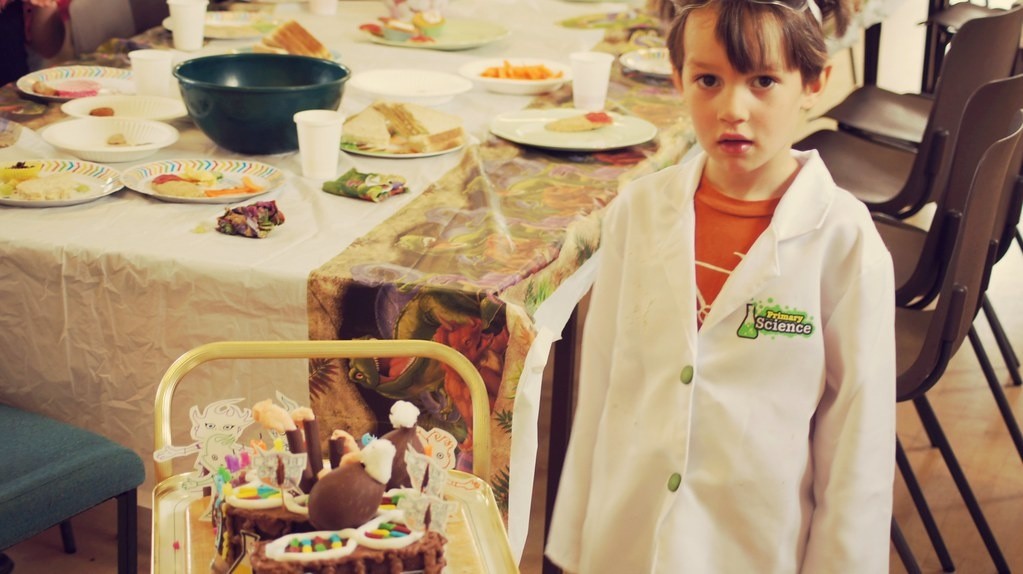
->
[122,158,288,201]
[1,115,22,147]
[226,45,352,71]
[341,117,466,159]
[490,109,657,152]
[348,65,472,109]
[0,159,125,207]
[162,11,286,39]
[16,66,135,98]
[42,117,180,163]
[619,48,674,78]
[457,58,573,94]
[355,19,509,50]
[60,93,190,123]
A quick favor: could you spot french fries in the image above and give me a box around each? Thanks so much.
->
[478,59,563,79]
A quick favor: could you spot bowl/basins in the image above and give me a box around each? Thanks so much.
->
[172,53,351,155]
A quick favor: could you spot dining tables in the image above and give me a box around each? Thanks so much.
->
[0,0,897,574]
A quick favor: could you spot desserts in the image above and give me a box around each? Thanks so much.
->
[381,9,445,42]
[201,399,448,574]
[0,161,69,198]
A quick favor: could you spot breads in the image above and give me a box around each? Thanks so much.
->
[252,18,330,59]
[338,101,466,155]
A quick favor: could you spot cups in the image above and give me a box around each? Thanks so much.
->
[128,49,193,117]
[166,0,210,50]
[570,52,615,110]
[293,110,346,179]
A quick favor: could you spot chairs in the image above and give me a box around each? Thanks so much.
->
[0,0,1023,574]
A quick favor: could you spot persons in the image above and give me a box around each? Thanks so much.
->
[23,0,169,70]
[545,0,896,574]
